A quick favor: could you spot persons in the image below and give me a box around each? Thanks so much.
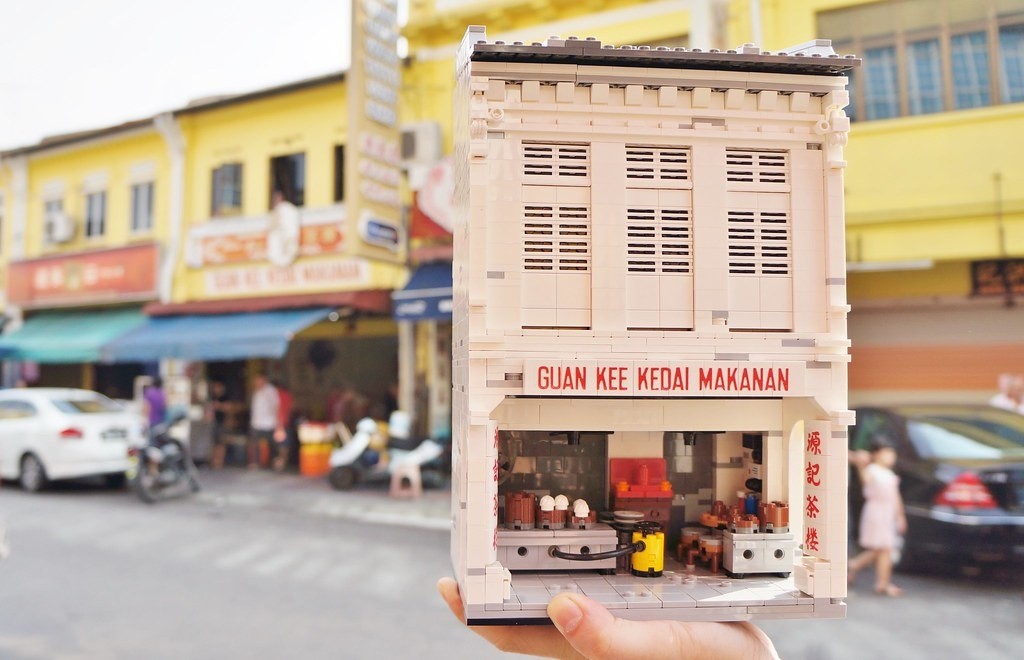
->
[438,575,781,660]
[847,439,908,597]
[137,376,167,445]
[206,370,400,472]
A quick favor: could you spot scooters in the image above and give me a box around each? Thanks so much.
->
[127,405,207,506]
[326,418,446,494]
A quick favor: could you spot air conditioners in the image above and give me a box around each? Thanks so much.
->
[43,214,76,243]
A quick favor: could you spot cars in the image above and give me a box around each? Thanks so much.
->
[848,400,1024,583]
[0,386,147,490]
